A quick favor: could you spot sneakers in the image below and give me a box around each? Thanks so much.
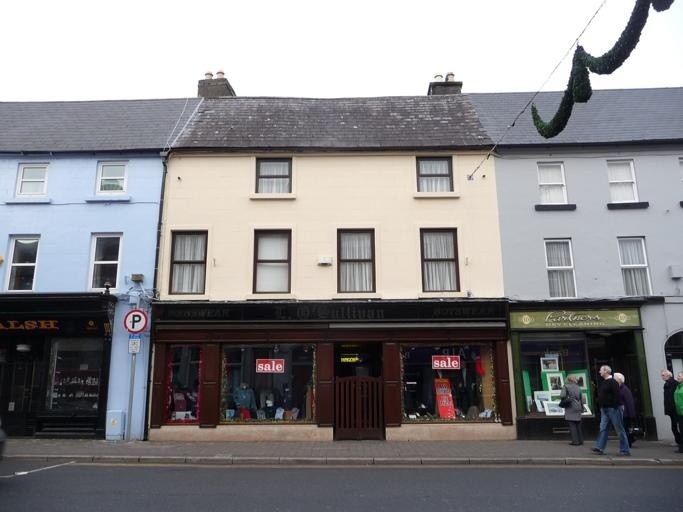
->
[674,448,683,453]
[569,441,583,446]
[591,447,601,453]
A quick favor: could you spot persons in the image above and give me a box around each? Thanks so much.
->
[661,370,680,446]
[673,370,683,453]
[591,365,632,456]
[233,382,257,410]
[613,373,636,448]
[560,376,583,445]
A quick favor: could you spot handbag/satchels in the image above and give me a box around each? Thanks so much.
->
[628,424,644,436]
[559,395,571,409]
